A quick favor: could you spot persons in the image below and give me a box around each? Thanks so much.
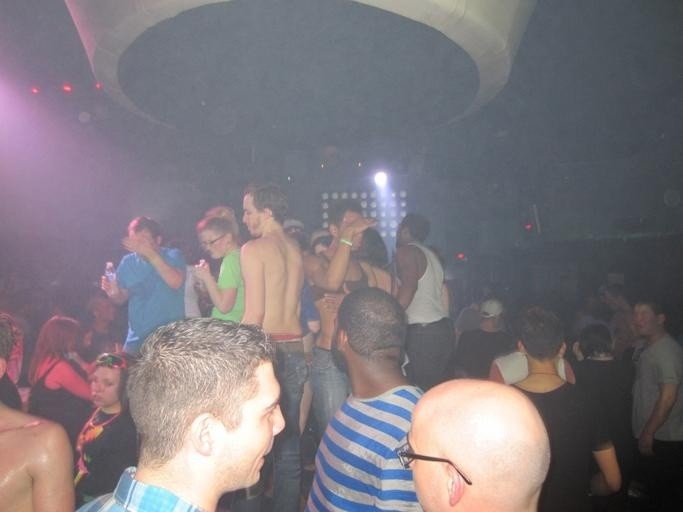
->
[0,181,681,511]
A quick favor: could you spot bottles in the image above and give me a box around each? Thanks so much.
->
[104,262,119,297]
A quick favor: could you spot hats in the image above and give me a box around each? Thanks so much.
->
[480,299,502,318]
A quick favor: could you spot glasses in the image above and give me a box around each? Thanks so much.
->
[200,234,222,247]
[396,444,474,484]
[96,352,127,370]
